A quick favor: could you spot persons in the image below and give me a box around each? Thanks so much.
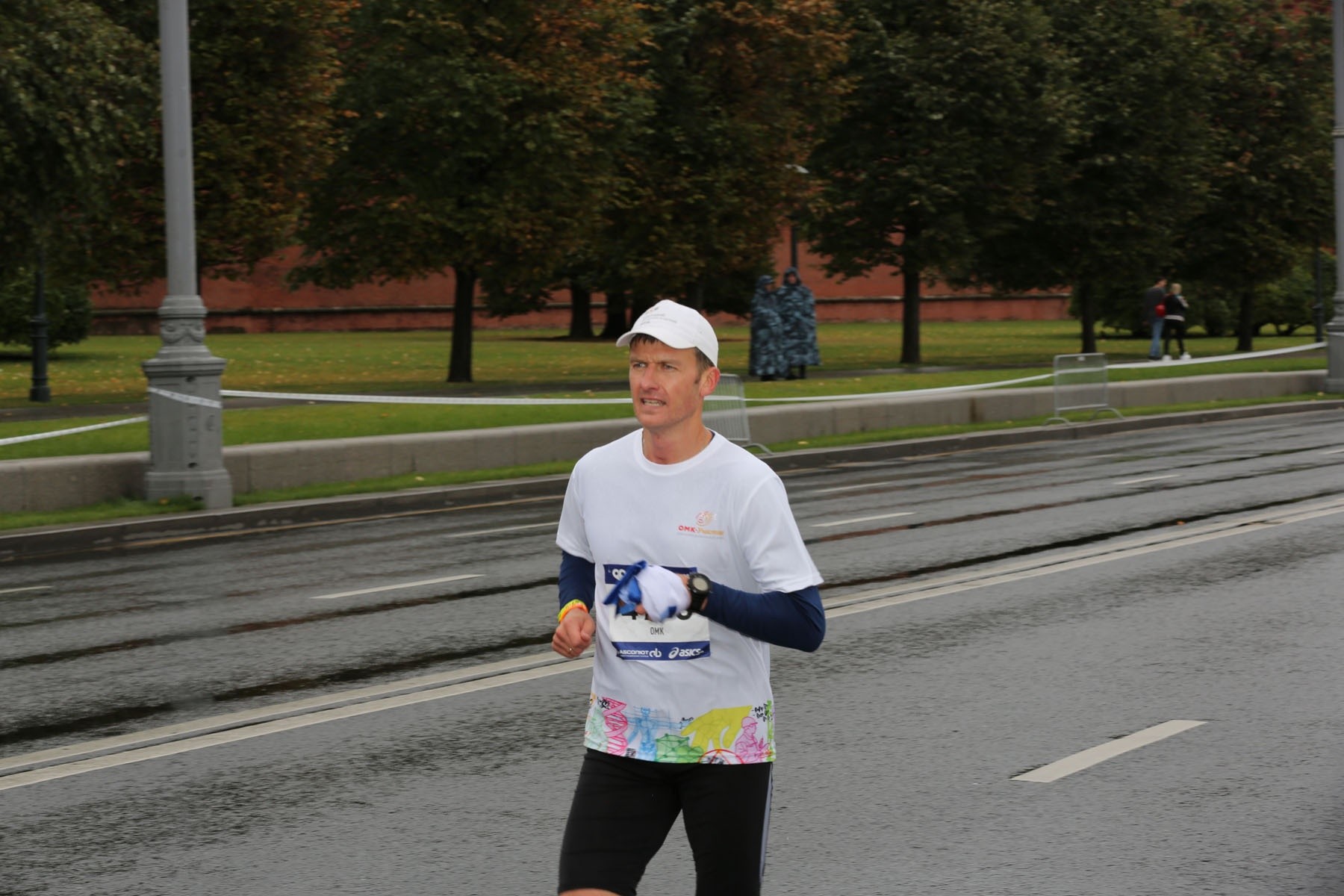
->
[552,298,825,896]
[1146,276,1192,361]
[751,267,822,382]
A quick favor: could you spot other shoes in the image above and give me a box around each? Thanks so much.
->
[1162,354,1173,360]
[1148,356,1161,360]
[1179,351,1191,359]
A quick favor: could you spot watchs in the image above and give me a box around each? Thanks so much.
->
[686,572,712,614]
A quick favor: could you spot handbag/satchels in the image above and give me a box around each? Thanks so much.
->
[1156,303,1165,317]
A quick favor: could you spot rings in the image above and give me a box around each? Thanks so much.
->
[568,647,572,655]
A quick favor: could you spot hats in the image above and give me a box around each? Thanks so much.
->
[756,276,777,286]
[617,298,719,368]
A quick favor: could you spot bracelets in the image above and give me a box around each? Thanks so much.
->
[557,598,588,624]
